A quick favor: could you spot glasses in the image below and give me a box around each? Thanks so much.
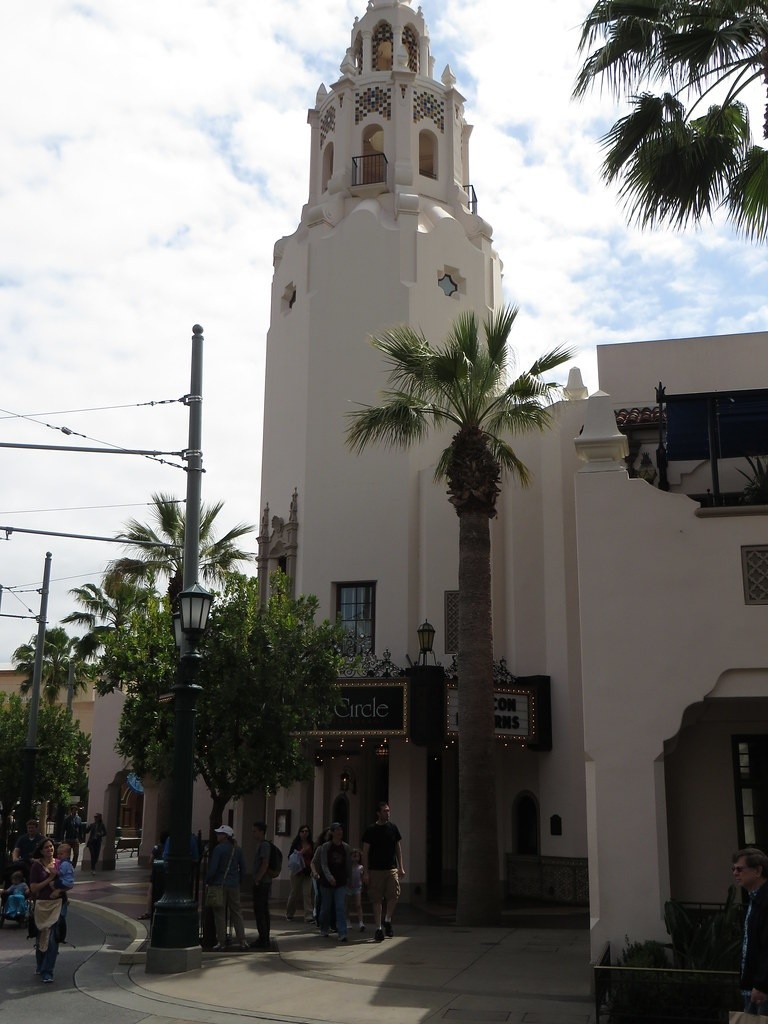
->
[301,830,308,833]
[732,866,757,872]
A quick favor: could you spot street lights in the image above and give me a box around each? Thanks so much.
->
[147,581,215,972]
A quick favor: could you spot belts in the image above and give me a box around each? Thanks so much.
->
[66,836,78,839]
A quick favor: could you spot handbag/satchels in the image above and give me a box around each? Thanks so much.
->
[729,1003,768,1024]
[289,851,305,876]
[205,885,225,907]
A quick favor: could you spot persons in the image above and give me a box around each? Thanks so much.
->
[204,825,250,950]
[136,845,162,919]
[345,848,367,932]
[732,847,768,1015]
[84,813,107,875]
[12,819,47,887]
[286,825,315,924]
[30,837,67,983]
[250,821,271,948]
[0,871,30,918]
[192,832,203,865]
[318,823,352,943]
[47,843,74,891]
[61,805,84,868]
[310,826,339,932]
[361,801,406,943]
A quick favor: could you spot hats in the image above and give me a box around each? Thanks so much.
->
[214,825,233,837]
[94,812,101,817]
[330,823,343,831]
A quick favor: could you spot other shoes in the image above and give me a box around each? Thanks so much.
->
[250,934,269,947]
[240,941,248,951]
[35,968,41,975]
[41,977,53,982]
[285,912,393,943]
[91,869,96,874]
[138,914,151,919]
[213,942,226,950]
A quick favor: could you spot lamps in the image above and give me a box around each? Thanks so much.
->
[373,742,389,760]
[340,771,357,796]
[415,618,438,667]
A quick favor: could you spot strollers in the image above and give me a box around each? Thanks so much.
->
[0,858,34,930]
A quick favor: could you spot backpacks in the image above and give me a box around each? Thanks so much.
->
[259,841,282,877]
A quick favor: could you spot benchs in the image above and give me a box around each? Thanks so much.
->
[115,838,141,859]
